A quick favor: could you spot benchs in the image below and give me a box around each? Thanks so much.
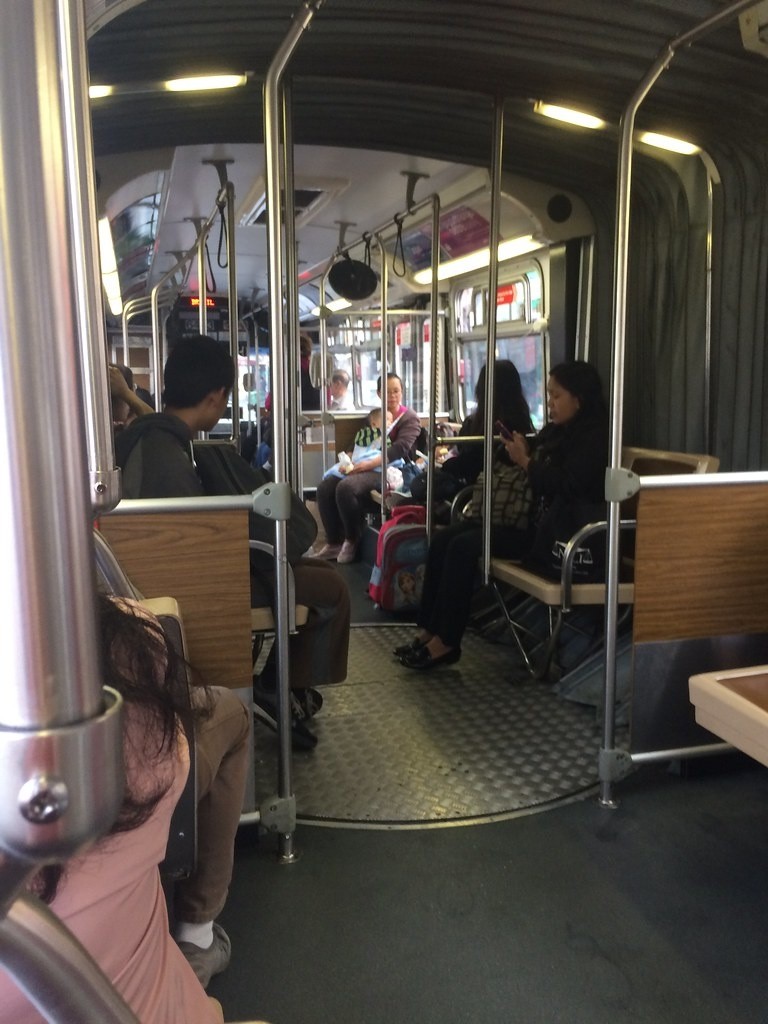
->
[369,422,720,683]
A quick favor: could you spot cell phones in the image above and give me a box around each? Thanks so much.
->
[494,421,514,441]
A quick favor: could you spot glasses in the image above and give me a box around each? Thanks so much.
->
[130,383,137,390]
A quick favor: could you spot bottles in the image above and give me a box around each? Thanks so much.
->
[440,448,453,463]
[338,451,354,474]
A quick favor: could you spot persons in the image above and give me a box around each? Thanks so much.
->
[332,370,354,410]
[352,408,427,474]
[110,335,352,750]
[0,591,259,1024]
[385,360,609,670]
[308,372,422,563]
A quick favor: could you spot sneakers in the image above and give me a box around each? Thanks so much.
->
[175,921,231,990]
[249,674,323,748]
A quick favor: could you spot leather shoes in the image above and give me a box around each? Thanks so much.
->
[392,636,431,655]
[400,645,462,668]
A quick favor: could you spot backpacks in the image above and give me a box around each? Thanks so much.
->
[369,512,429,612]
[187,444,318,569]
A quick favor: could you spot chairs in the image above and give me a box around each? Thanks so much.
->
[249,540,318,753]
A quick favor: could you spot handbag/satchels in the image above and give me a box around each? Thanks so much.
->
[410,469,460,503]
[342,442,387,475]
[472,456,540,530]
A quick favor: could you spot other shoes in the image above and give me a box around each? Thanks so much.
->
[337,540,358,563]
[309,544,341,560]
